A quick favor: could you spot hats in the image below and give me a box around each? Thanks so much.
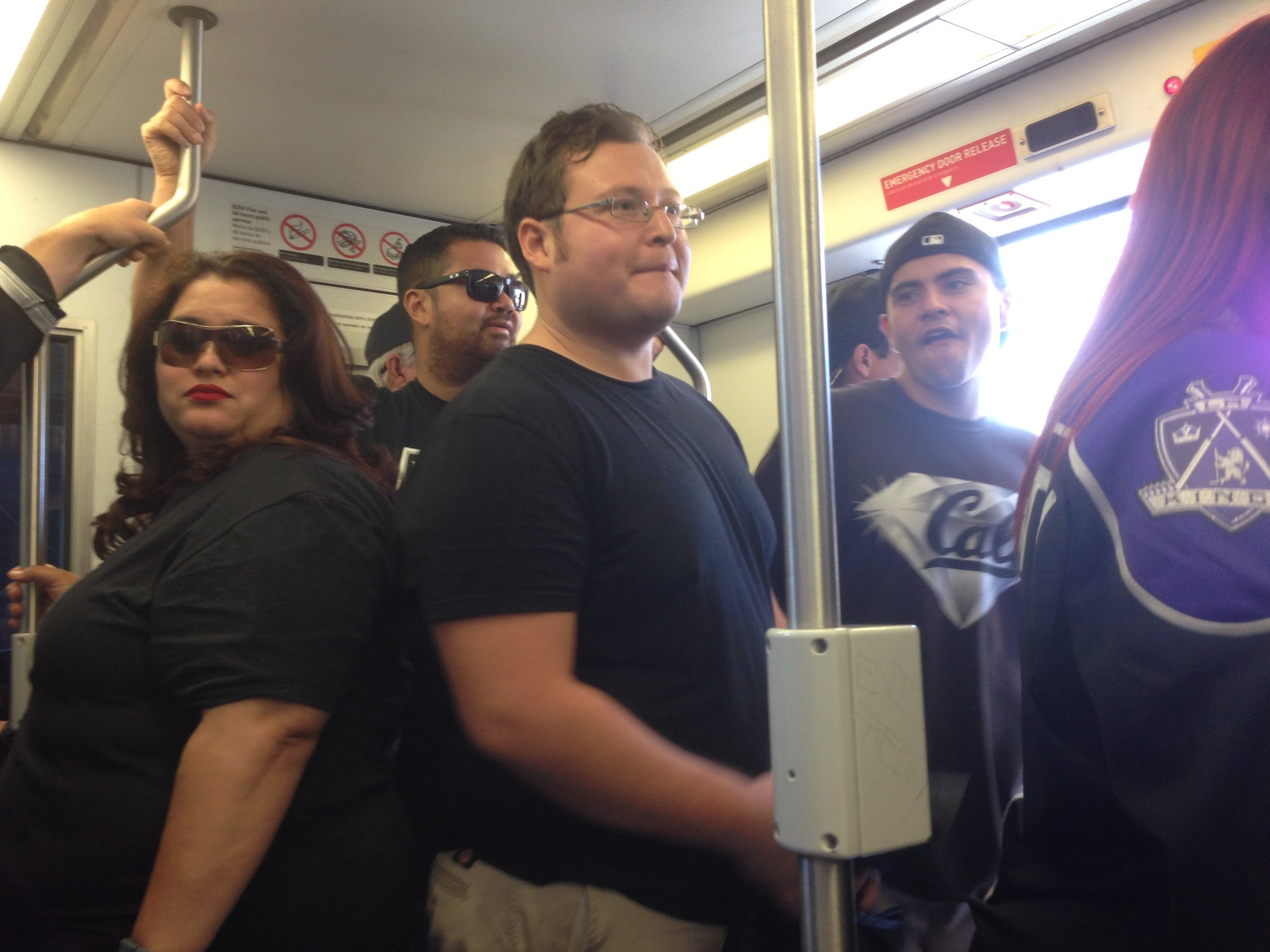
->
[829,273,887,384]
[365,302,413,366]
[882,210,1006,290]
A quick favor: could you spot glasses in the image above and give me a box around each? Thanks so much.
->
[544,195,705,229]
[152,318,292,371]
[417,269,528,311]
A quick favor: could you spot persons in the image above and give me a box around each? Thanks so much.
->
[1012,12,1270,952]
[0,76,1041,952]
